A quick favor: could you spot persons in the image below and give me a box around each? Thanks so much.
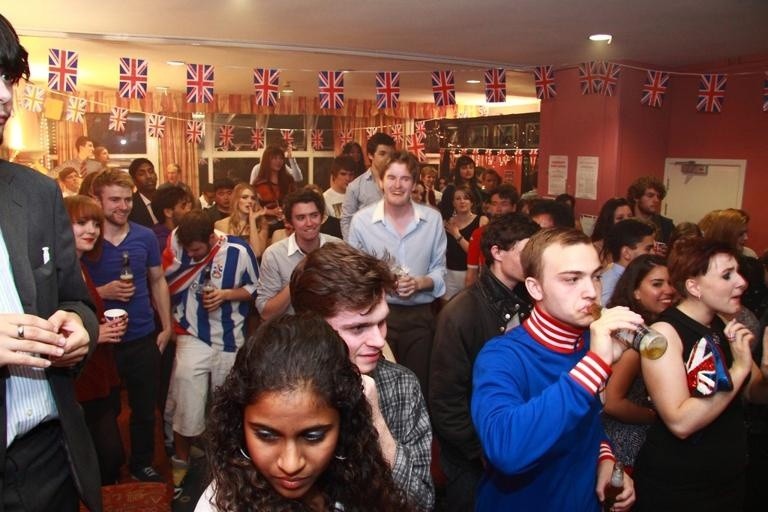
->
[56,133,767,511]
[1,11,100,510]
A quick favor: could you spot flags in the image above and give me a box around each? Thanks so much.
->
[485,68,506,101]
[108,106,128,131]
[147,106,539,168]
[641,69,668,108]
[597,61,620,95]
[23,85,46,112]
[49,47,78,91]
[697,74,727,113]
[66,97,85,122]
[579,62,598,95]
[186,64,214,104]
[119,57,147,98]
[431,70,456,105]
[535,66,555,98]
[376,72,400,109]
[319,72,345,109]
[253,68,279,106]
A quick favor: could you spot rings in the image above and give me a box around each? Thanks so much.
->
[18,328,25,340]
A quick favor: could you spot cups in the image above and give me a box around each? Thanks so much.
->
[391,272,408,296]
[654,242,666,252]
[104,308,125,339]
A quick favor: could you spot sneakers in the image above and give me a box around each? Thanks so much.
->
[129,463,167,482]
[168,455,191,500]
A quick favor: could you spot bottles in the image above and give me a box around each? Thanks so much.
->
[604,461,625,512]
[119,251,134,284]
[588,304,668,360]
[201,267,214,305]
